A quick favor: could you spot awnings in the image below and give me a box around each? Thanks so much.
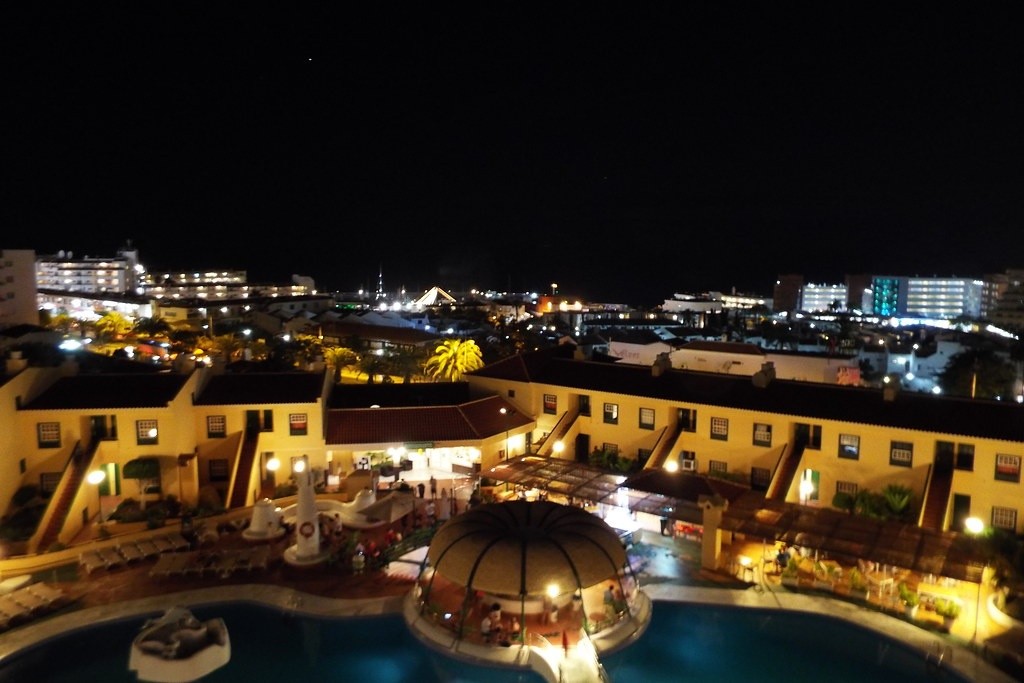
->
[121,456,176,479]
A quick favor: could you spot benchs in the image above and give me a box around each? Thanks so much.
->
[380,522,445,566]
[430,601,471,636]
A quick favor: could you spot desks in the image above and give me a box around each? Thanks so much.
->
[590,612,607,634]
[917,582,951,606]
[865,572,894,599]
[820,559,843,583]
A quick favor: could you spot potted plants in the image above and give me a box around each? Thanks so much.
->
[781,557,800,587]
[933,598,960,632]
[849,566,869,600]
[813,562,841,591]
[898,581,920,618]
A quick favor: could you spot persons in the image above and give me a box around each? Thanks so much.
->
[424,500,438,526]
[279,516,292,536]
[345,527,403,558]
[468,490,481,509]
[479,602,521,647]
[539,490,548,501]
[430,476,438,501]
[660,505,670,536]
[602,584,626,616]
[416,483,425,499]
[777,545,791,573]
[333,513,343,545]
[516,489,526,501]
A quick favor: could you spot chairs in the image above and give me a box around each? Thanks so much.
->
[118,542,140,564]
[166,552,191,581]
[97,547,123,570]
[732,555,761,584]
[135,539,160,557]
[149,552,176,579]
[0,581,66,629]
[761,539,777,576]
[151,534,173,556]
[80,550,105,574]
[166,532,190,554]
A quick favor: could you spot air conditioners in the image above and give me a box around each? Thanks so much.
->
[682,459,697,472]
